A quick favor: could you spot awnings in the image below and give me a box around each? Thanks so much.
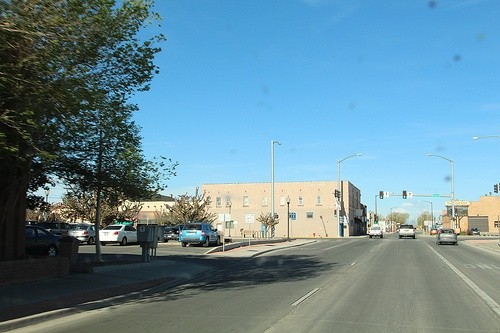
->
[355,218,362,223]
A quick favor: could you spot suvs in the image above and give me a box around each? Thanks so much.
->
[368,225,383,239]
[178,222,220,248]
[399,224,417,239]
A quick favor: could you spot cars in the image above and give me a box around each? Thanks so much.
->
[30,221,103,246]
[435,228,458,245]
[162,228,178,243]
[25,225,60,258]
[468,227,481,235]
[98,224,138,246]
[430,228,438,235]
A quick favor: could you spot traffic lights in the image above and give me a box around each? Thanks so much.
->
[403,190,407,199]
[337,191,341,198]
[379,191,383,199]
[454,208,458,216]
[493,183,498,193]
[335,190,338,198]
[498,182,500,192]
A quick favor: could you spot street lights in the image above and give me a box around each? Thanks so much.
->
[270,140,282,236]
[391,205,401,232]
[420,200,433,228]
[228,201,233,238]
[286,196,290,243]
[338,152,363,236]
[425,153,456,230]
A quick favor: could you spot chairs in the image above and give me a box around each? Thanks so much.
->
[24,232,32,237]
